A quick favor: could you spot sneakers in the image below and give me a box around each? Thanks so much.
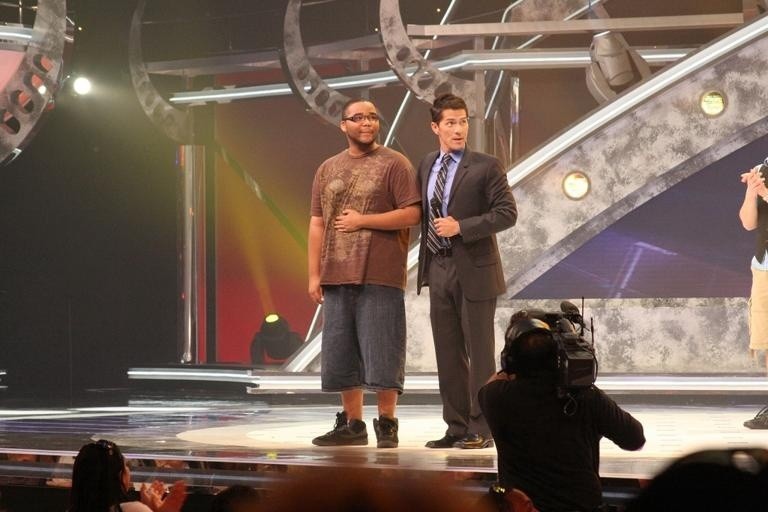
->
[373,415,399,450]
[742,406,767,430]
[311,408,370,449]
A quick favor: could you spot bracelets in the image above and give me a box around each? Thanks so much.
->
[762,196,767,201]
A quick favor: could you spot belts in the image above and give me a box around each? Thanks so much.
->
[439,249,454,257]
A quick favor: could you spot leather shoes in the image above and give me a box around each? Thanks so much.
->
[452,431,494,450]
[424,431,466,449]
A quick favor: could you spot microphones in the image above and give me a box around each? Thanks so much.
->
[430,197,452,249]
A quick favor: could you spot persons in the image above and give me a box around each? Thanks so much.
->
[63,437,768,511]
[477,307,646,511]
[737,155,768,430]
[416,92,520,451]
[305,97,423,449]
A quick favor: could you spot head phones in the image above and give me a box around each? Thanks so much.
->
[501,318,553,374]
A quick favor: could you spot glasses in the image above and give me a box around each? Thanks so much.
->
[343,113,379,124]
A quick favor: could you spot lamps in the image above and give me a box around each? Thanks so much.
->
[250,314,302,365]
[582,31,654,105]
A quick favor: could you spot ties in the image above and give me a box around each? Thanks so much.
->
[426,155,452,254]
